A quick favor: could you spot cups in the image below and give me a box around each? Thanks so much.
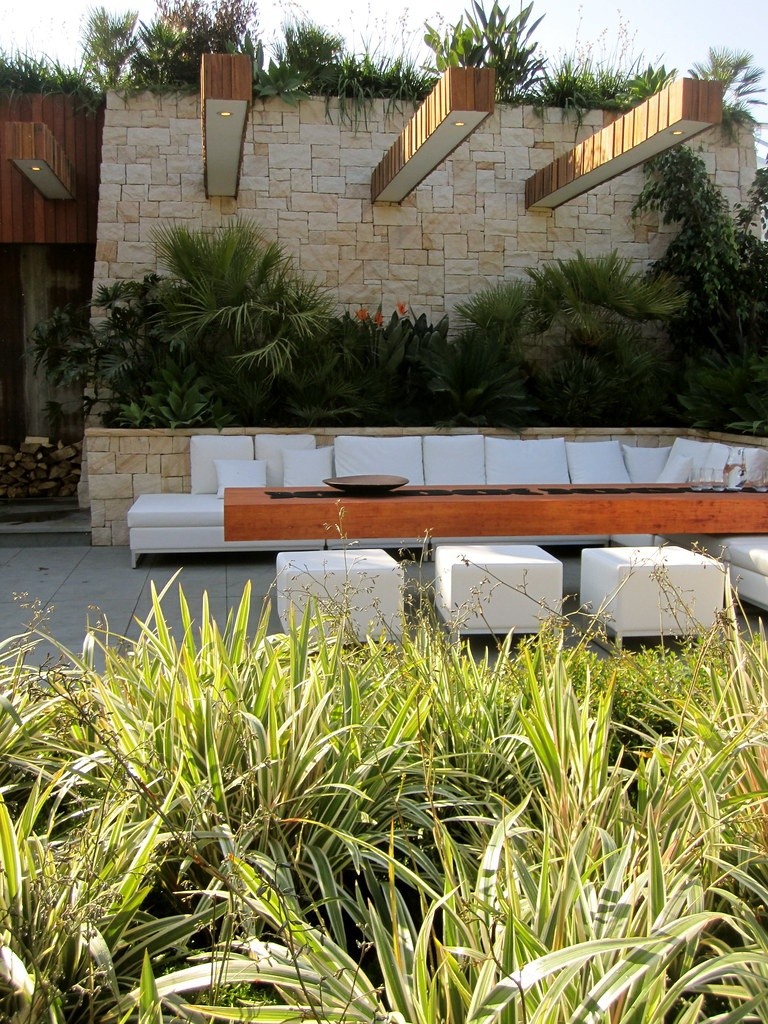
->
[688,466,768,492]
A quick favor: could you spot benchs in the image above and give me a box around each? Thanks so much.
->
[653,531,768,611]
[126,492,325,568]
[610,533,653,548]
[327,539,429,562]
[430,535,610,562]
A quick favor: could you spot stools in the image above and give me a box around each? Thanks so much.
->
[578,546,725,656]
[435,545,563,651]
[275,549,407,650]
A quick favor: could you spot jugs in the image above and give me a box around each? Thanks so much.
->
[722,446,746,491]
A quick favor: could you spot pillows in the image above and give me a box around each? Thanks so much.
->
[335,437,423,486]
[659,438,711,484]
[281,449,332,487]
[214,461,267,499]
[190,436,254,495]
[485,437,570,485]
[622,444,671,484]
[256,435,315,487]
[424,436,485,487]
[566,441,630,484]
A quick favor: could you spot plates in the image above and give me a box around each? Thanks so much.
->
[323,474,409,492]
